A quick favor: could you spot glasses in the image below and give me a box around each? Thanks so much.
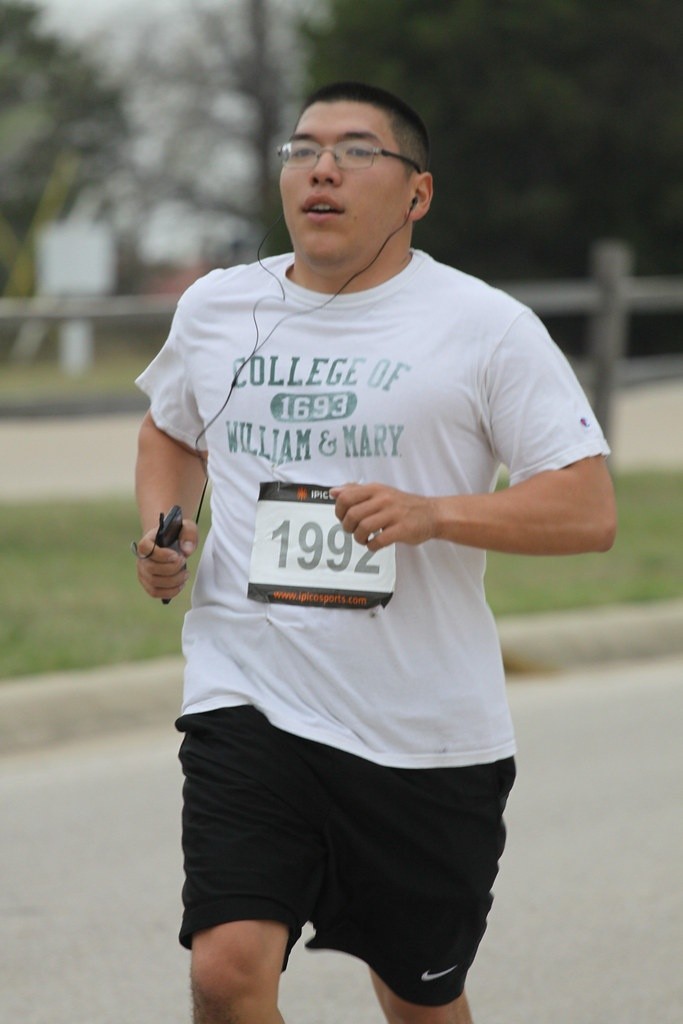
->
[275,139,423,174]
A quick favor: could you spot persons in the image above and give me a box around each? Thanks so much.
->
[132,75,623,1024]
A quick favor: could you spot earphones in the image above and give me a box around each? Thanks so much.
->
[409,197,418,213]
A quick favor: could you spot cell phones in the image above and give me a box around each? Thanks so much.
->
[156,505,186,604]
